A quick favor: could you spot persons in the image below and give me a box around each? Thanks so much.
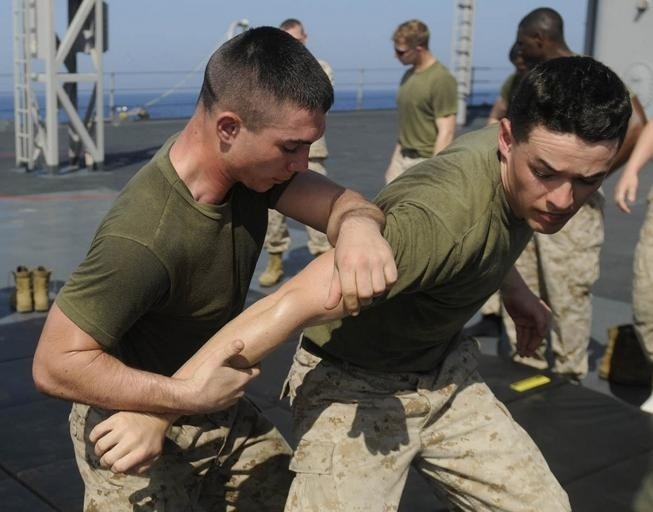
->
[255,14,336,285]
[496,7,647,390]
[487,41,540,134]
[618,117,652,361]
[28,26,399,512]
[87,55,634,512]
[386,19,460,185]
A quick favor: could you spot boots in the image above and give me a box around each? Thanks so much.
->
[599,324,653,384]
[9,266,51,312]
[259,254,283,286]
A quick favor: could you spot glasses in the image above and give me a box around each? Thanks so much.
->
[395,48,411,56]
